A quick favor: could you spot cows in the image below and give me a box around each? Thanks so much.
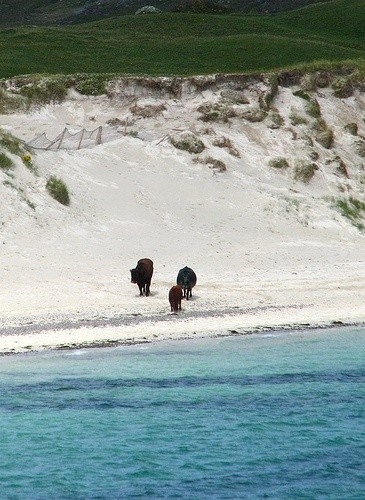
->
[169,285,183,313]
[130,258,153,297]
[177,266,197,300]
